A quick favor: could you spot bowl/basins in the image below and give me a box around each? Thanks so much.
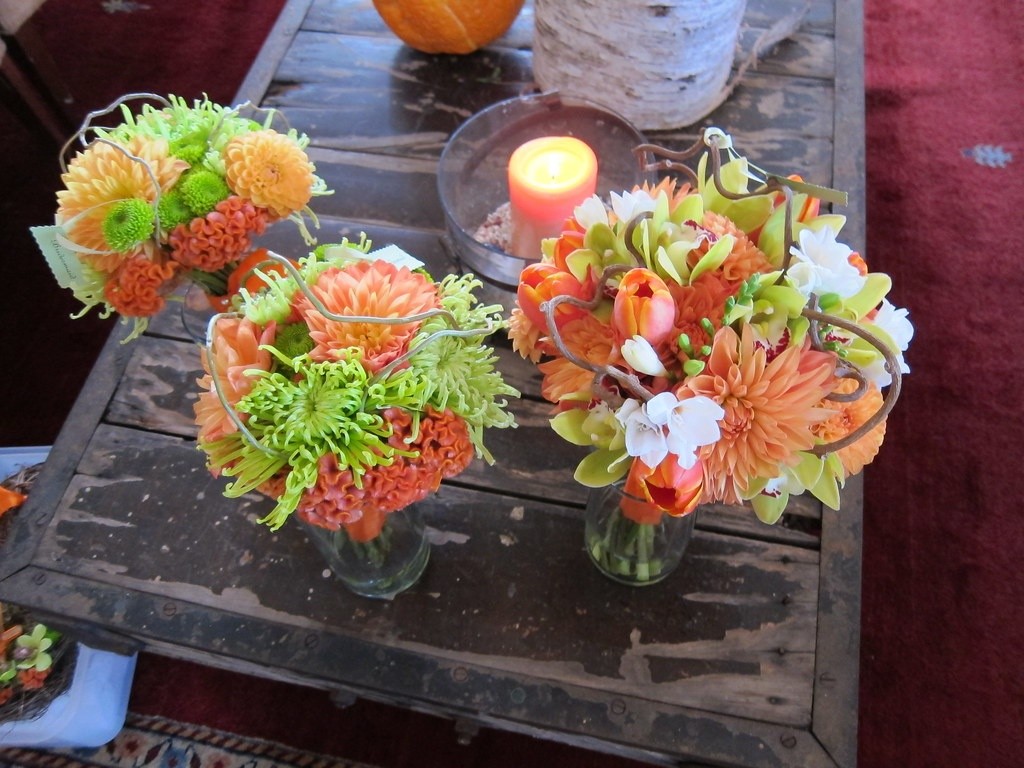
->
[438,93,656,287]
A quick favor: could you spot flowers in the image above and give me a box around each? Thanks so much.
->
[0,463,55,708]
[192,232,522,532]
[27,91,335,345]
[503,126,914,527]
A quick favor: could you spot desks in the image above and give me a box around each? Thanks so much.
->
[0,0,868,768]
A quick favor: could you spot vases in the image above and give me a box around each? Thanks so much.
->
[180,250,253,347]
[585,480,697,587]
[296,504,432,601]
